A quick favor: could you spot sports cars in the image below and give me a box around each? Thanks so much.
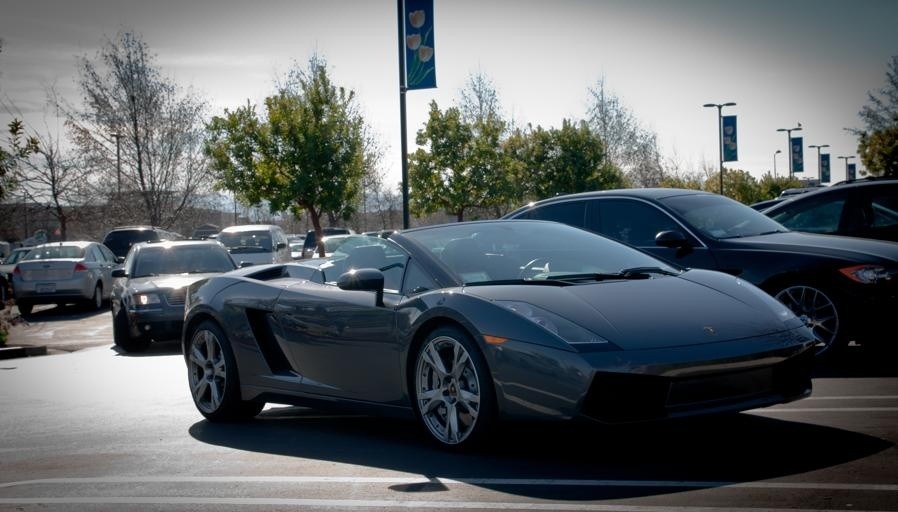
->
[181,219,820,454]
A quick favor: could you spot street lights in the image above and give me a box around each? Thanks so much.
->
[108,133,127,200]
[704,102,858,196]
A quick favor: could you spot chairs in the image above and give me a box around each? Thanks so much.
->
[343,243,391,287]
[439,237,493,289]
